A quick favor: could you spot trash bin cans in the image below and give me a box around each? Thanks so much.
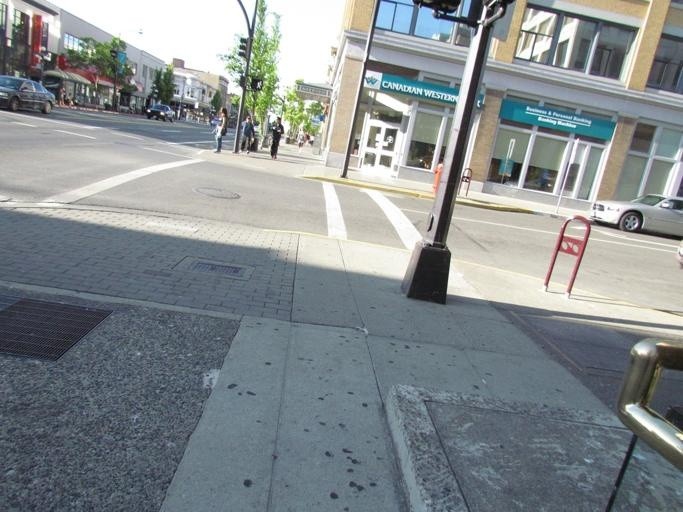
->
[250,138,259,151]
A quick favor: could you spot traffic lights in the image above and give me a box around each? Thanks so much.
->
[238,37,246,56]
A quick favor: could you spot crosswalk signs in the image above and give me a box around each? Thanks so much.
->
[251,79,262,90]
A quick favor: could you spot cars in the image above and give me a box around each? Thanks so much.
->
[588,193,682,238]
[0,74,55,114]
[145,104,175,122]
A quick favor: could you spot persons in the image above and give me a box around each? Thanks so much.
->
[58,82,66,107]
[67,92,73,110]
[210,106,228,154]
[239,115,255,154]
[294,126,315,154]
[270,116,284,161]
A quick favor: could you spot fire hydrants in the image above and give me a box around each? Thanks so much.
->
[431,163,442,194]
